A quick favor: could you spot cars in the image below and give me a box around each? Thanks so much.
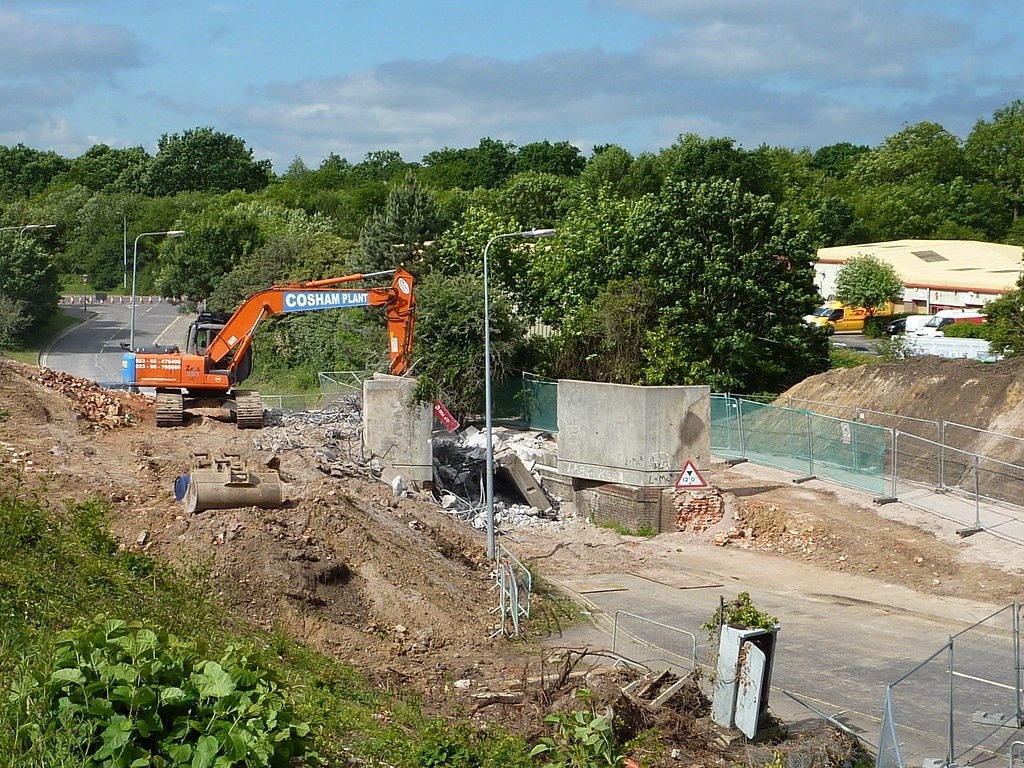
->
[880,318,906,334]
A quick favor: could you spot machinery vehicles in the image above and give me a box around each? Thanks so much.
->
[119,267,416,429]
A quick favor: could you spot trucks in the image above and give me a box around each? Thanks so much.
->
[802,297,894,336]
[905,316,943,336]
[914,309,988,338]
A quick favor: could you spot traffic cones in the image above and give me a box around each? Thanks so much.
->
[78,295,83,304]
[119,295,123,303]
[148,297,153,304]
[110,296,114,304]
[129,295,133,304]
[70,295,75,304]
[138,296,144,304]
[89,295,93,304]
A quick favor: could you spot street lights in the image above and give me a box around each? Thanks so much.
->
[130,231,186,352]
[117,212,126,289]
[484,229,558,561]
[20,225,57,241]
